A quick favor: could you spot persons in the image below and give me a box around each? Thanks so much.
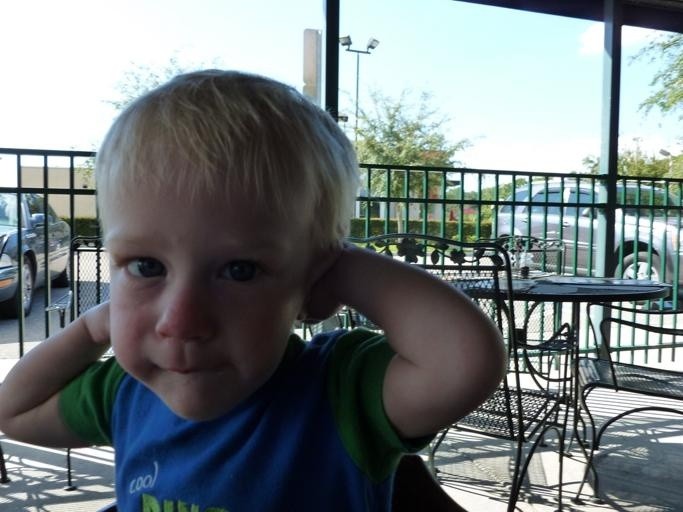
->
[0,68,508,512]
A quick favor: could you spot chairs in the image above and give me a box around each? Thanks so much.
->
[0,236,112,493]
[650,343,653,346]
[337,232,600,511]
[474,235,566,420]
[566,302,683,511]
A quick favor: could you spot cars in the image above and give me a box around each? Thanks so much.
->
[0,189,72,320]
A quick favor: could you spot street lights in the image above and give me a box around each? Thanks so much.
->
[338,35,381,155]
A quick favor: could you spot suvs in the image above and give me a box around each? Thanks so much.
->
[488,177,682,315]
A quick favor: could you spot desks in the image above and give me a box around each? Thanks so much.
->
[405,278,670,511]
